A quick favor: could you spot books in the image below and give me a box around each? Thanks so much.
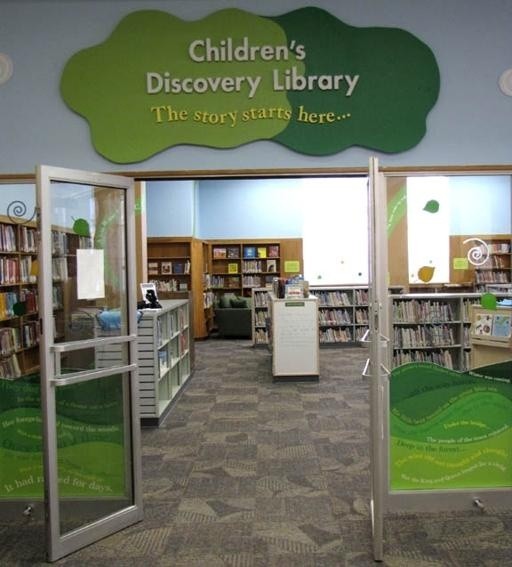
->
[201,246,309,344]
[309,288,457,372]
[463,244,512,371]
[1,224,93,379]
[148,260,190,370]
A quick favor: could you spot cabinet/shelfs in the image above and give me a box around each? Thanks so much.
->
[147,235,210,340]
[1,214,97,384]
[204,237,305,329]
[387,293,474,373]
[95,298,193,429]
[310,282,474,349]
[448,232,512,369]
[249,286,271,347]
